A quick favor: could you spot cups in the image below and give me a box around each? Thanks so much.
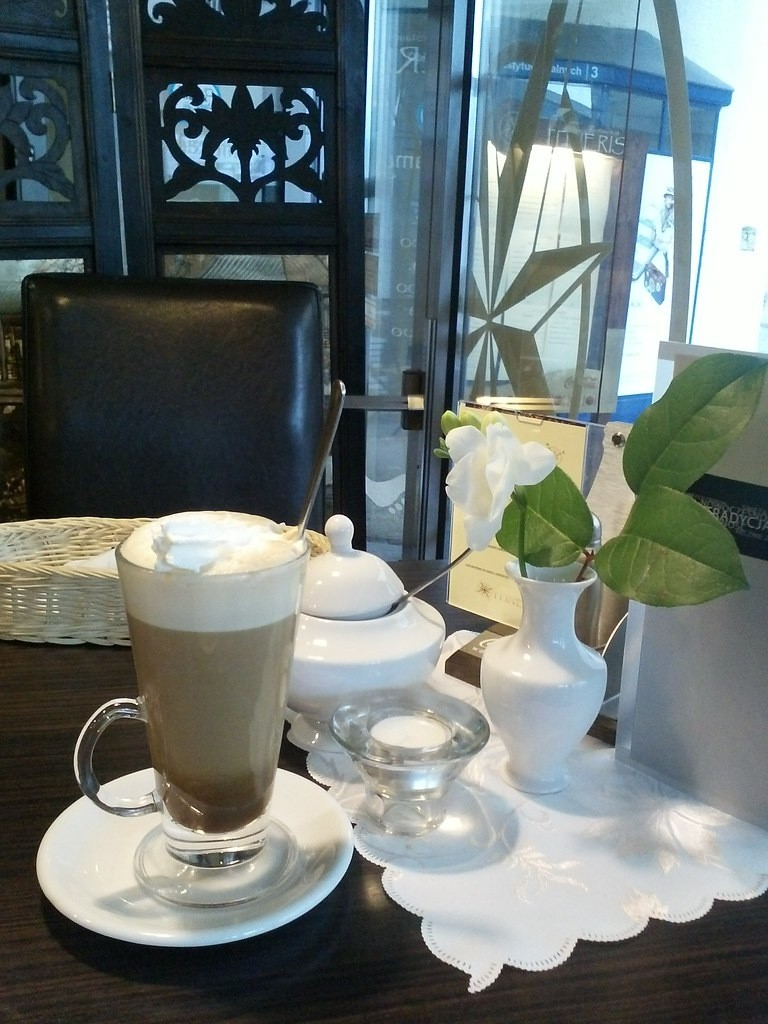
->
[72,528,310,910]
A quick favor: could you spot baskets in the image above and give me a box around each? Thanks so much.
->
[0,514,332,647]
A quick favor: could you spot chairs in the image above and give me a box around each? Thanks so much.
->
[20,272,348,532]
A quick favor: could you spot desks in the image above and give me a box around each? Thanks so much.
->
[0,557,768,1024]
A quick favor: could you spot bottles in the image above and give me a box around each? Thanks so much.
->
[285,514,447,754]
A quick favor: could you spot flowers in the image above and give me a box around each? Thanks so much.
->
[431,352,768,608]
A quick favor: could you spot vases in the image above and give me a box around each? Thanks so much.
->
[481,560,609,793]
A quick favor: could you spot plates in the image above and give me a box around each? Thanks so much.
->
[36,768,356,948]
